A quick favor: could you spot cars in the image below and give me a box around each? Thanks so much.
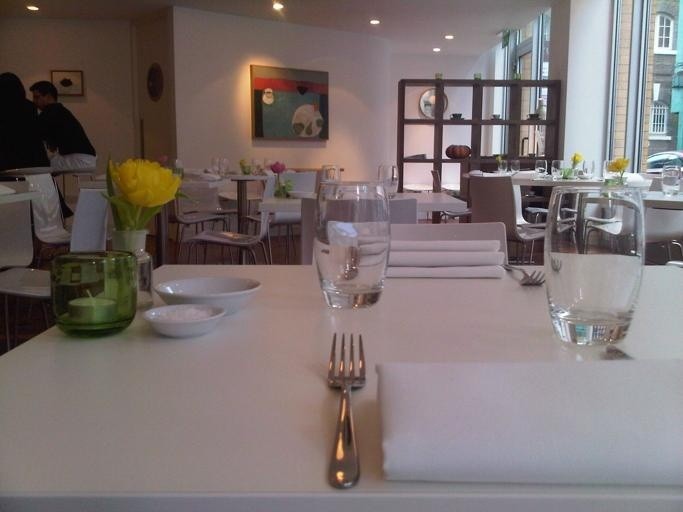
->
[643,152,683,177]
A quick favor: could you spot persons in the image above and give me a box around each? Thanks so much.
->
[29,80,96,157]
[0,72,75,223]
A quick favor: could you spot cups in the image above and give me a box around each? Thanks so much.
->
[316,161,400,198]
[542,187,647,348]
[527,114,538,118]
[499,159,682,198]
[313,182,394,309]
[450,114,461,119]
[492,115,499,119]
[213,152,279,177]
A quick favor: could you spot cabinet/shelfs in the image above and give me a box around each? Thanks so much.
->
[396,78,561,224]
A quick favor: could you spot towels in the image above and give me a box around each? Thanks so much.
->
[374,358,683,487]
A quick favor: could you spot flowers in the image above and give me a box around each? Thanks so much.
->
[563,152,584,179]
[606,158,630,185]
[239,158,247,170]
[100,151,198,231]
[270,161,295,198]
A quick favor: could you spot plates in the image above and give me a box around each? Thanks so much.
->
[491,118,501,120]
[451,118,464,120]
[526,117,539,120]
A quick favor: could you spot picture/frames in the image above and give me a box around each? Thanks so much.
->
[50,69,84,97]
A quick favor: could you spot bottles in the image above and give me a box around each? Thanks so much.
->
[537,96,546,120]
[103,250,154,308]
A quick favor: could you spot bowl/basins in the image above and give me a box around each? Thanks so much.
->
[51,250,137,339]
[141,304,226,339]
[152,275,264,313]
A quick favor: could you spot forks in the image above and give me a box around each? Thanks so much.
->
[327,330,371,492]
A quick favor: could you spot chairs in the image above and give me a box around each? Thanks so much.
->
[1,165,683,351]
